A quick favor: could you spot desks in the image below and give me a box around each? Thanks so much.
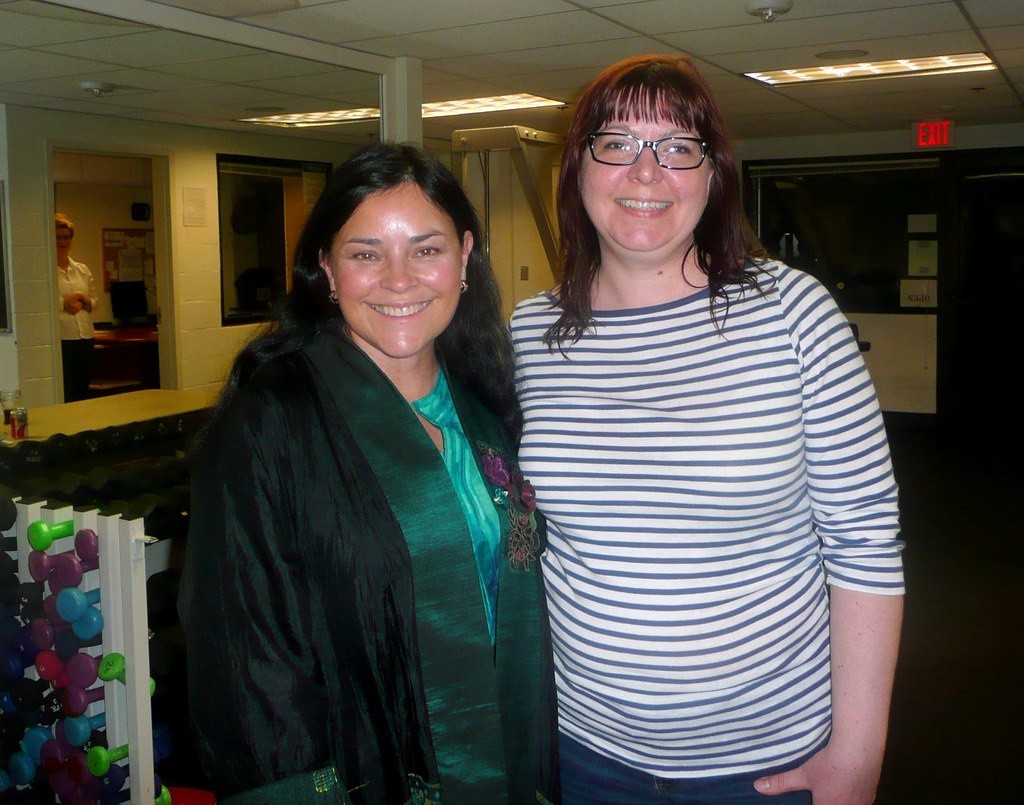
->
[0,389,220,442]
[94,329,157,345]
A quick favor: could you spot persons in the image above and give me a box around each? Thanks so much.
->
[56,213,97,402]
[507,56,907,804]
[172,142,562,805]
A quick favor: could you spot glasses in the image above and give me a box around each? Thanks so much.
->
[55,234,72,240]
[583,131,714,170]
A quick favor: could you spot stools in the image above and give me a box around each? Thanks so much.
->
[90,379,139,389]
